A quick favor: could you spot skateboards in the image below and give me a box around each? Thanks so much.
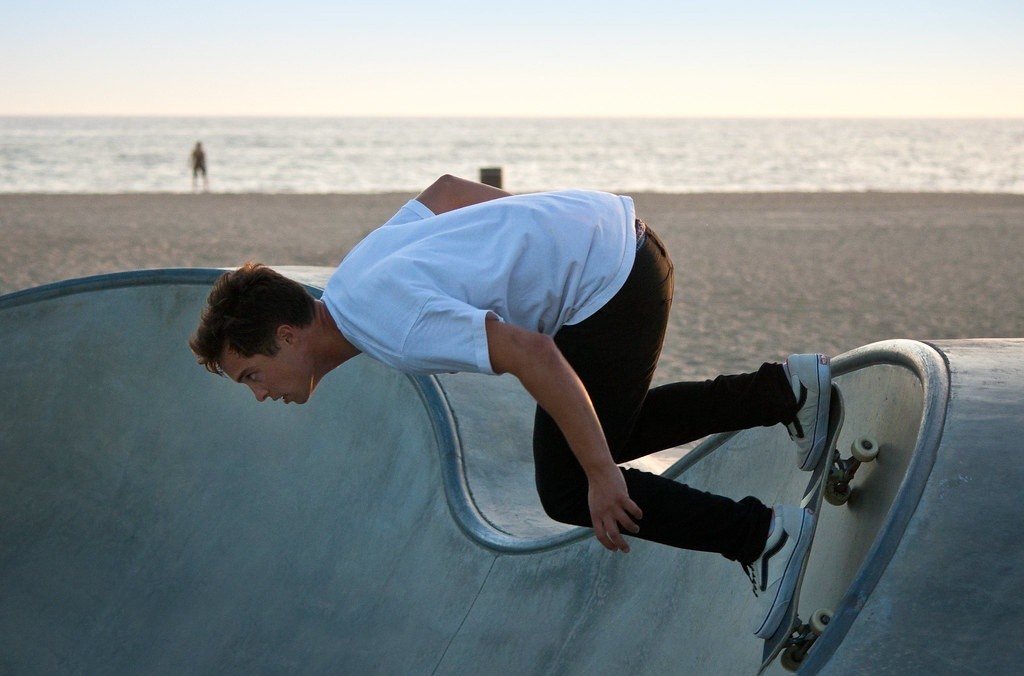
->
[753,380,879,676]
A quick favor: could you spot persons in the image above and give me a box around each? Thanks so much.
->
[191,175,832,641]
[192,142,207,177]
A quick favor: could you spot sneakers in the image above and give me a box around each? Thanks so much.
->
[782,353,831,472]
[741,500,817,641]
